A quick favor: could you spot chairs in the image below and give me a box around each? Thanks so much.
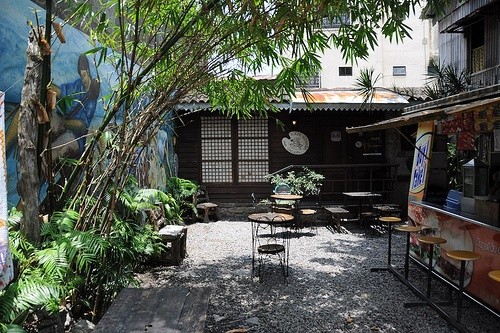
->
[299,183,321,234]
[251,215,291,285]
[191,184,220,223]
[251,192,272,213]
[146,200,187,265]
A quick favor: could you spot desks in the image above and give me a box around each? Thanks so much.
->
[90,286,210,333]
[248,212,296,273]
[342,192,382,221]
[270,194,303,237]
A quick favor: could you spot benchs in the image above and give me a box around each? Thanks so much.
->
[370,217,500,333]
[323,203,402,235]
